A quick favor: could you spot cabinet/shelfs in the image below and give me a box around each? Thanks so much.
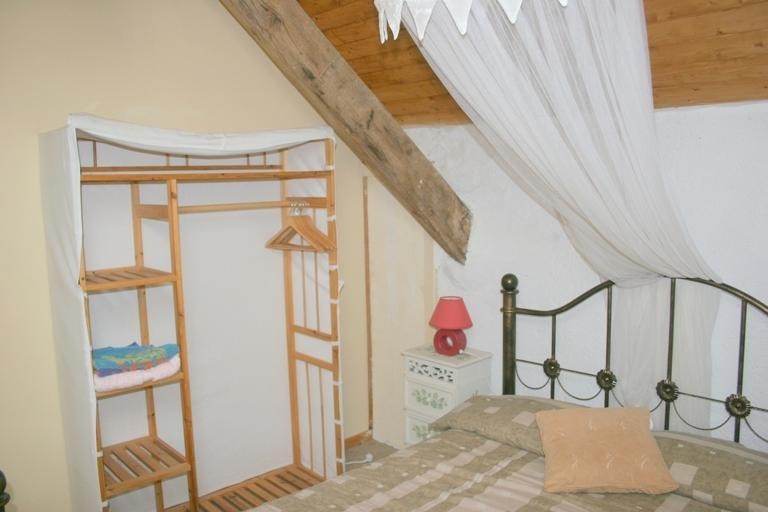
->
[31,111,356,512]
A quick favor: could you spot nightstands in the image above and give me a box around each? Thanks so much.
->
[399,343,494,446]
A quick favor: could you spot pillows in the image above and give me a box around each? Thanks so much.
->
[534,399,679,502]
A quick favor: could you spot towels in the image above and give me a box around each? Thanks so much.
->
[89,337,181,392]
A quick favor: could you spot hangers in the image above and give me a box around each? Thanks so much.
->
[262,197,335,253]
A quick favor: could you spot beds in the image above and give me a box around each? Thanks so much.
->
[237,271,768,512]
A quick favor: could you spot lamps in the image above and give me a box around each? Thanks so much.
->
[427,294,475,357]
